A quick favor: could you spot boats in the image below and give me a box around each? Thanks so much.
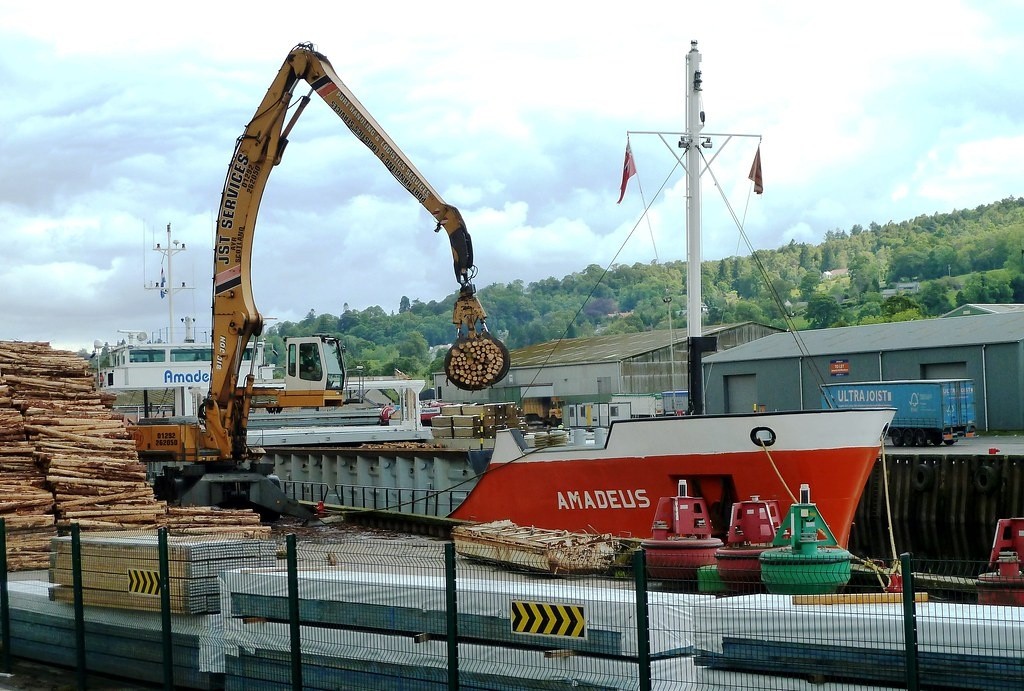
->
[86,39,899,553]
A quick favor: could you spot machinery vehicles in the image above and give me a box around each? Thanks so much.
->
[127,39,509,524]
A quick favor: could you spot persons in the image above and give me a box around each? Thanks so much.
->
[100,373,104,387]
[303,350,321,375]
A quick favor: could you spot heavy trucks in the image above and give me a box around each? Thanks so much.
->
[819,380,978,447]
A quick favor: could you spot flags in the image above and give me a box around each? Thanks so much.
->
[616,143,636,205]
[160,268,166,299]
[748,147,763,194]
